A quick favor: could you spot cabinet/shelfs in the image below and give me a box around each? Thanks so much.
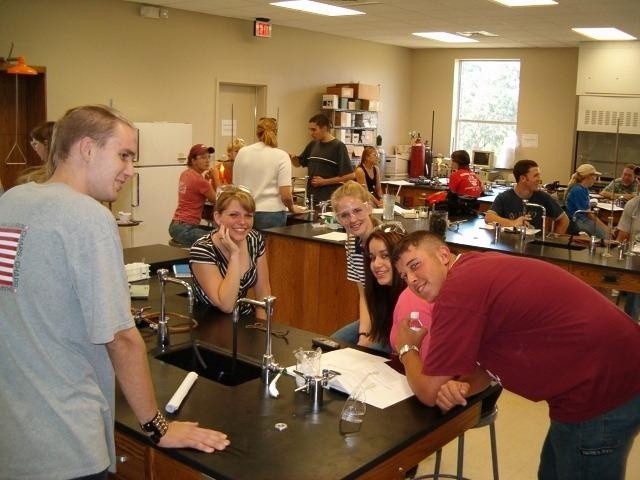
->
[0,65,47,193]
[327,109,378,161]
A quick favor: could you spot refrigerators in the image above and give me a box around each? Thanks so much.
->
[112,122,192,248]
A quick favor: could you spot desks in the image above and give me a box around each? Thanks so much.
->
[114,177,639,480]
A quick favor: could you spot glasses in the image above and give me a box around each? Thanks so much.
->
[215,184,253,201]
[334,202,370,227]
[378,221,406,238]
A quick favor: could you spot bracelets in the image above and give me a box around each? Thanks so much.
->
[397,345,421,363]
[356,333,371,338]
[139,410,169,446]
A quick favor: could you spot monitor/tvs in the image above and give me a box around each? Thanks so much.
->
[472,150,494,172]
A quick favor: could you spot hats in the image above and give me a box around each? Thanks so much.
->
[188,143,215,160]
[574,163,601,179]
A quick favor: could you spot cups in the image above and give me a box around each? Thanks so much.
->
[383,195,395,220]
[429,210,448,240]
[295,352,322,388]
[118,212,131,222]
[557,188,564,200]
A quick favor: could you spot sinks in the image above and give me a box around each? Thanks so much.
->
[148,339,269,387]
[529,240,587,251]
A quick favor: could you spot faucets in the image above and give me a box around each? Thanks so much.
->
[321,201,331,213]
[519,198,546,240]
[234,294,287,399]
[293,174,309,207]
[573,209,602,255]
[152,267,209,347]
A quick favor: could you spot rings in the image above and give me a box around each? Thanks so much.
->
[219,237,224,240]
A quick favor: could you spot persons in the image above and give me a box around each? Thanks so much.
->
[169,143,220,246]
[1,104,231,480]
[363,221,410,352]
[212,138,245,193]
[388,286,436,360]
[232,117,304,230]
[15,121,56,183]
[432,149,640,321]
[187,184,273,321]
[355,146,385,208]
[289,113,358,217]
[389,229,640,479]
[330,179,387,352]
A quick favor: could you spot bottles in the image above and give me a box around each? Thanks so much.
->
[408,311,422,331]
[619,196,624,207]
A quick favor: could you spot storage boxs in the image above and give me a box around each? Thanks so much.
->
[322,83,381,160]
[384,145,410,181]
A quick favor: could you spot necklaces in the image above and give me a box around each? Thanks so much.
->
[449,252,463,270]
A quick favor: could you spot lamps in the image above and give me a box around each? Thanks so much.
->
[6,40,38,75]
[140,4,171,22]
